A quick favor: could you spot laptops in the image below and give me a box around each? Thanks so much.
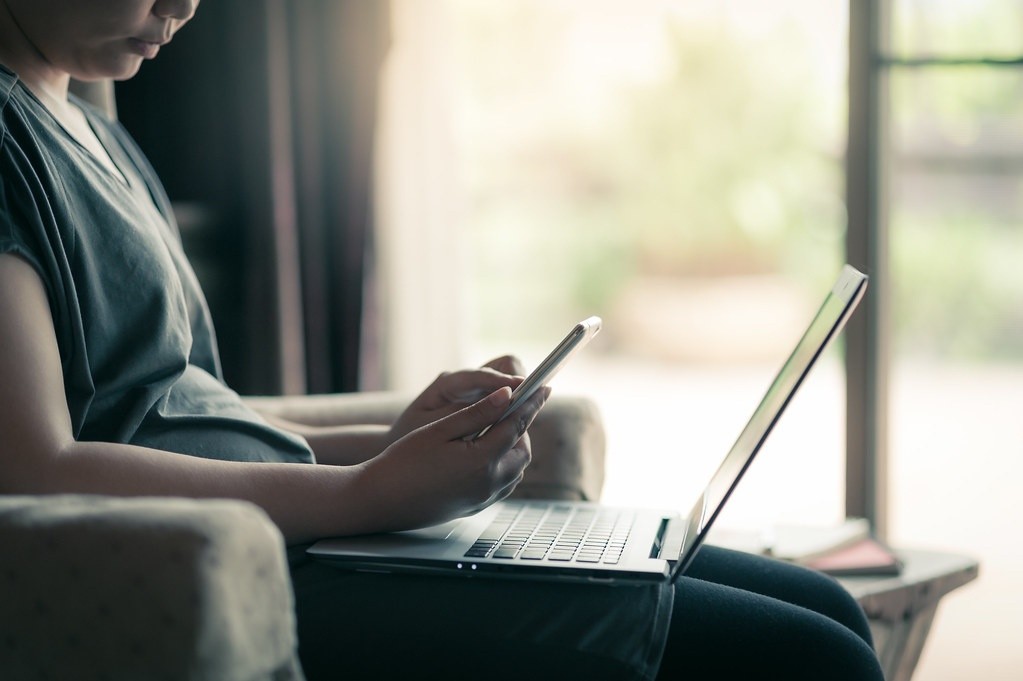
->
[301,265,870,587]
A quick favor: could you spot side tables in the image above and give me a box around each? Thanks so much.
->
[836,542,983,681]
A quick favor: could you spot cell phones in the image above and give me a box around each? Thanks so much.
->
[473,315,601,443]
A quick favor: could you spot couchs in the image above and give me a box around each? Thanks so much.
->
[0,392,605,681]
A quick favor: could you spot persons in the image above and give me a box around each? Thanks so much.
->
[0,0,888,681]
